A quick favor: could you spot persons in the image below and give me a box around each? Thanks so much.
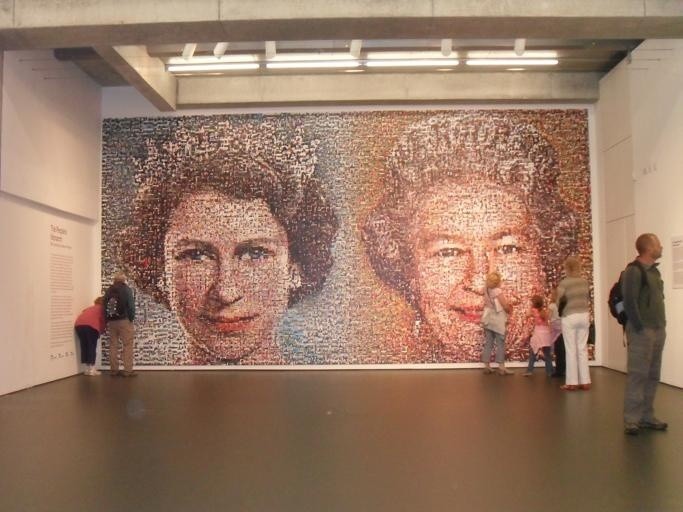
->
[112,122,339,363]
[607,233,666,433]
[73,295,105,376]
[103,273,138,375]
[546,288,566,377]
[360,115,579,363]
[523,296,554,377]
[553,257,592,389]
[478,244,514,375]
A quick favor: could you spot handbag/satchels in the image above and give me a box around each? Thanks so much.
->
[587,321,596,345]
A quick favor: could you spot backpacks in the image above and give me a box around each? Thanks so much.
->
[608,260,647,327]
[103,285,126,320]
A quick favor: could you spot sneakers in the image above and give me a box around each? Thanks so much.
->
[81,367,138,377]
[638,416,668,430]
[622,421,641,434]
[481,364,590,392]
